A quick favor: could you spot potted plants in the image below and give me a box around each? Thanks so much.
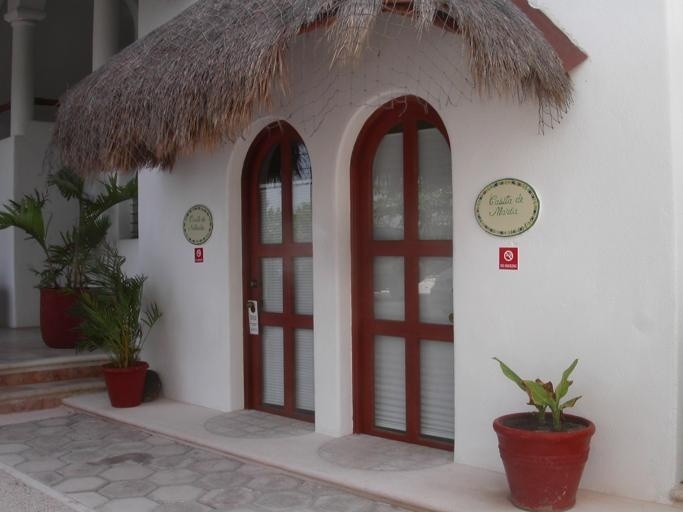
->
[0,169,138,348]
[71,241,150,407]
[491,357,595,512]
